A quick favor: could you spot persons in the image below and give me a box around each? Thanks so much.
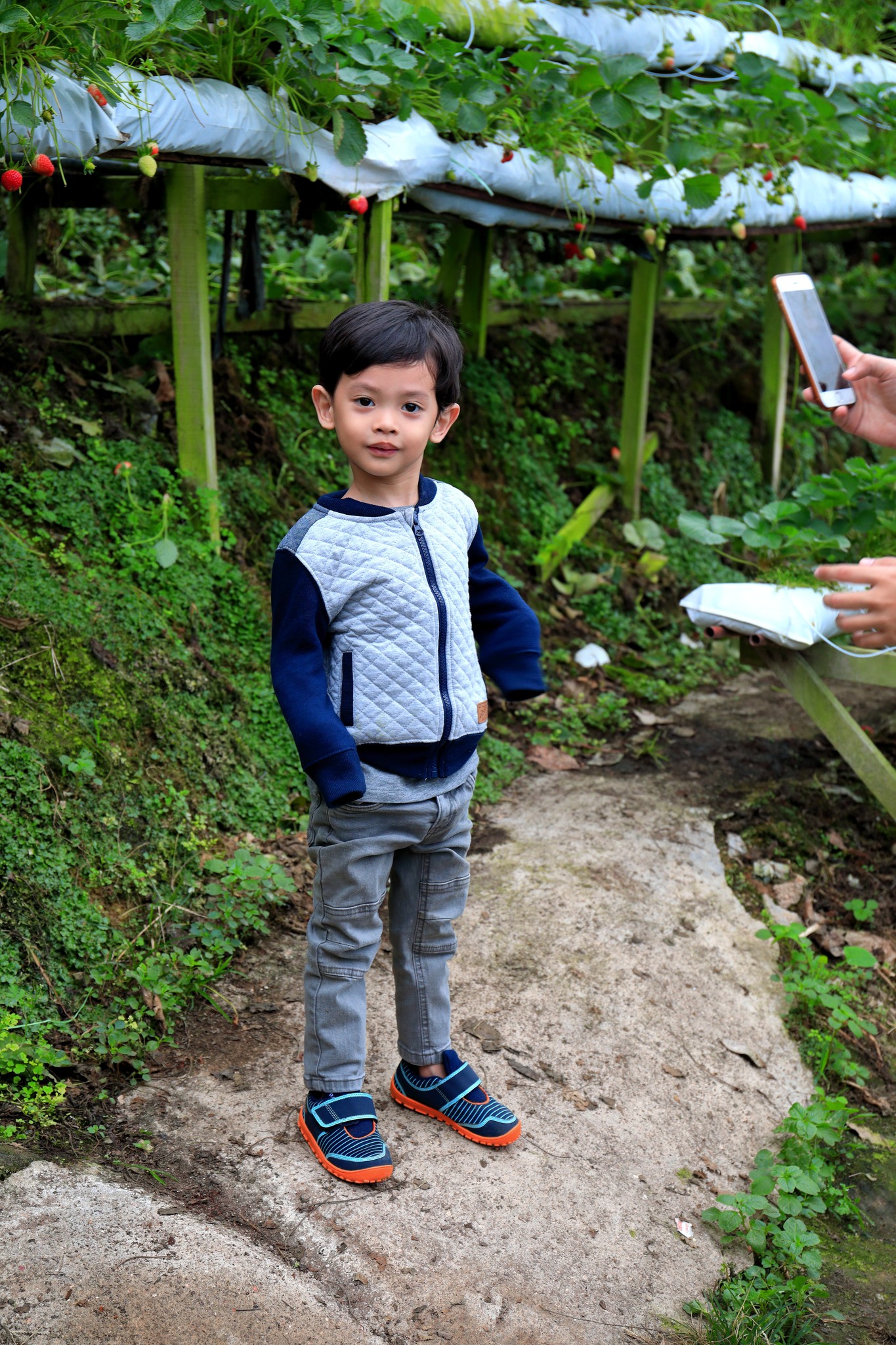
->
[273,301,549,1185]
[798,334,896,649]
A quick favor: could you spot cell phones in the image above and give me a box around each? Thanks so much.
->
[770,271,856,410]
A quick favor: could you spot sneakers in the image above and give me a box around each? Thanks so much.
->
[390,1048,523,1146]
[298,1093,392,1182]
